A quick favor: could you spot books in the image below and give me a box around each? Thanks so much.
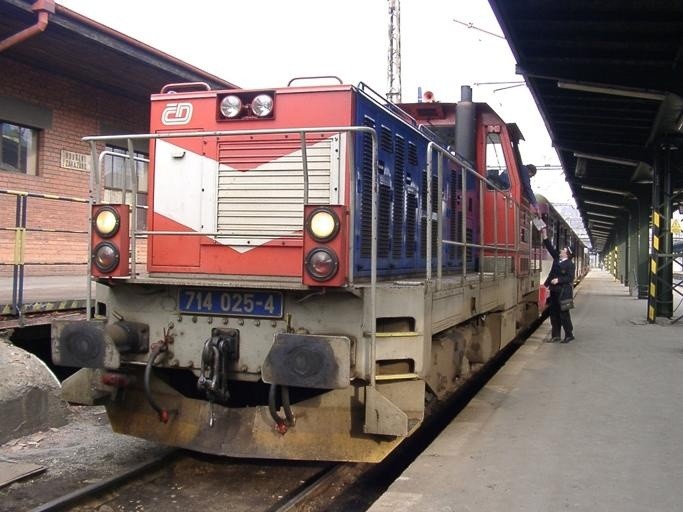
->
[531,217,547,232]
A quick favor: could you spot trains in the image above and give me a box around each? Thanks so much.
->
[46,75,597,469]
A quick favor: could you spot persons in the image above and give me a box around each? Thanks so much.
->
[498,164,543,220]
[541,228,579,343]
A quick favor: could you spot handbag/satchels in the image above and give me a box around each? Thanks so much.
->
[558,297,575,312]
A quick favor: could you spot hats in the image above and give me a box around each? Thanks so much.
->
[567,246,576,257]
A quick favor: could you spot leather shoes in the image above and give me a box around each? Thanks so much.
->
[560,334,575,344]
[547,335,561,343]
